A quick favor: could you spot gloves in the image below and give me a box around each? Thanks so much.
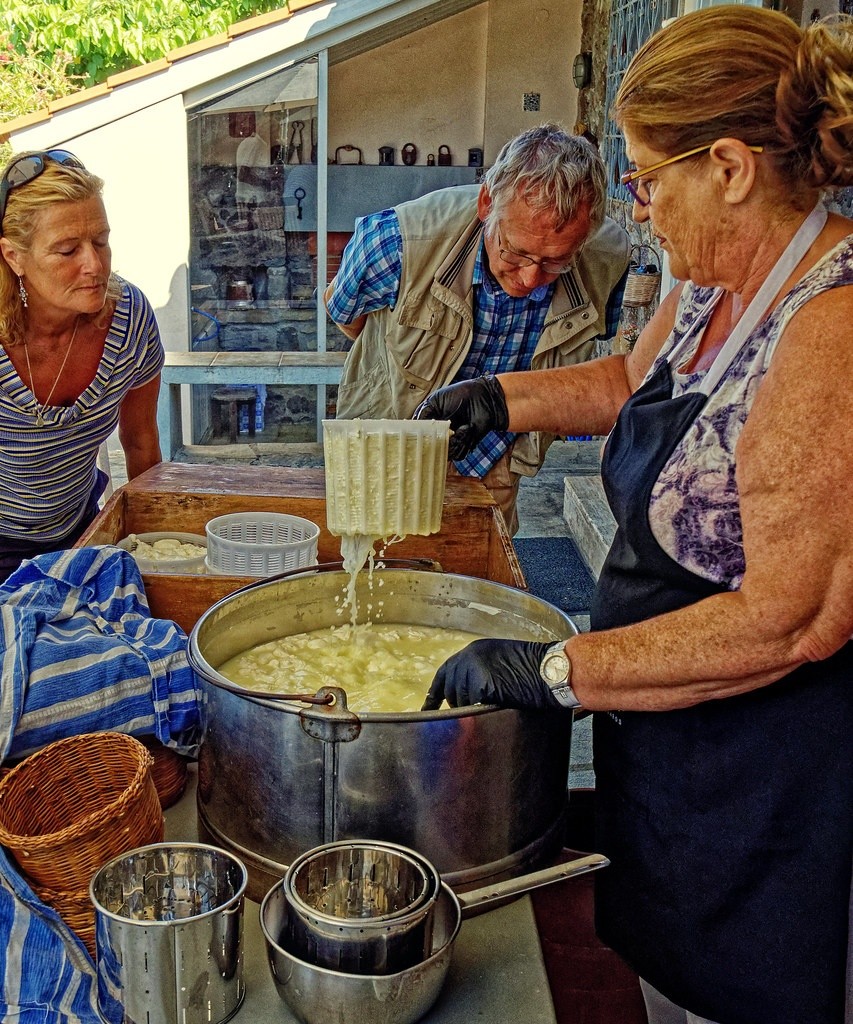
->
[420,638,563,716]
[411,374,509,462]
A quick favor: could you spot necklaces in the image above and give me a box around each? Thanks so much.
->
[23,320,81,427]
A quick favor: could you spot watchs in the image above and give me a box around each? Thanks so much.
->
[539,643,583,709]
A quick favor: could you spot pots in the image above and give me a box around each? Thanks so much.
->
[259,852,611,1024]
[186,556,583,920]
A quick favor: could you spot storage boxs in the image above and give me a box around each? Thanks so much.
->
[61,463,528,639]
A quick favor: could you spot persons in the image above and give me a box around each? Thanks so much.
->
[1,150,164,589]
[407,4,852,1024]
[316,125,635,544]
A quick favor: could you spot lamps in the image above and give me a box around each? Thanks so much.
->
[572,51,592,89]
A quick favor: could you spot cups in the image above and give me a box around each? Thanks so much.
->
[89,843,249,1024]
[283,839,440,975]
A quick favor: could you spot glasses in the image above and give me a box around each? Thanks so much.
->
[0,149,85,237]
[496,221,588,273]
[620,146,763,207]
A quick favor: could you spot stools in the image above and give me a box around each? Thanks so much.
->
[209,387,260,440]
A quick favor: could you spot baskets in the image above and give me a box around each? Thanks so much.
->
[622,243,662,307]
[321,419,451,535]
[0,733,187,963]
[116,512,320,577]
